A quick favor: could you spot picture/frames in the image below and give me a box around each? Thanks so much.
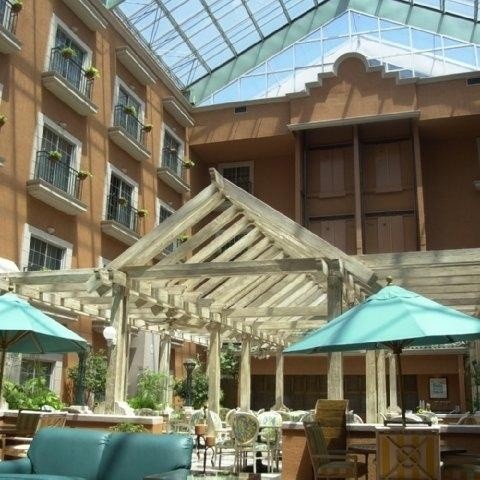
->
[428,376,449,400]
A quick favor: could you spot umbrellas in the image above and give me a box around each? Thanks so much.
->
[279,273,480,427]
[0,288,89,403]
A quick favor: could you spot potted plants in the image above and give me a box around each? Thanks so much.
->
[139,124,153,135]
[84,65,101,80]
[59,47,78,62]
[183,159,194,170]
[116,196,127,206]
[169,149,178,155]
[133,208,148,218]
[76,169,91,181]
[2,114,9,126]
[10,1,24,15]
[124,105,137,117]
[48,151,62,161]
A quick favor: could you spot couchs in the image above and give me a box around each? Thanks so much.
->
[0,428,191,480]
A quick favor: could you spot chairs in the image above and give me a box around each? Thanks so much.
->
[1,411,49,435]
[1,414,66,458]
[191,400,479,480]
